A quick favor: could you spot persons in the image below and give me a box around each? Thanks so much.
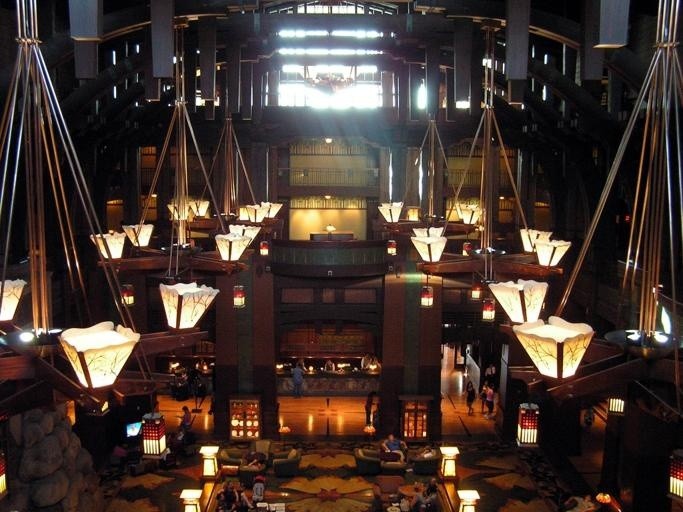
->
[563,495,595,512]
[480,381,489,415]
[179,406,192,430]
[462,380,477,416]
[486,383,496,416]
[583,407,596,434]
[405,445,434,473]
[417,478,440,512]
[214,451,268,512]
[484,363,496,377]
[290,358,337,399]
[381,434,408,461]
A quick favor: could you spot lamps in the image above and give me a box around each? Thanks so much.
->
[87,17,260,312]
[501,0,683,504]
[168,63,287,256]
[179,489,202,512]
[457,489,480,512]
[199,446,219,481]
[439,446,460,477]
[410,21,571,324]
[0,0,220,500]
[375,111,477,256]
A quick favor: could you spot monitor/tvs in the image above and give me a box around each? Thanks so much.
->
[123,421,142,438]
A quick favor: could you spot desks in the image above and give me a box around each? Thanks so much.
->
[399,483,426,499]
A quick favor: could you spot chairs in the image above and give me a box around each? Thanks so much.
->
[353,440,441,475]
[373,484,392,510]
[220,440,302,472]
